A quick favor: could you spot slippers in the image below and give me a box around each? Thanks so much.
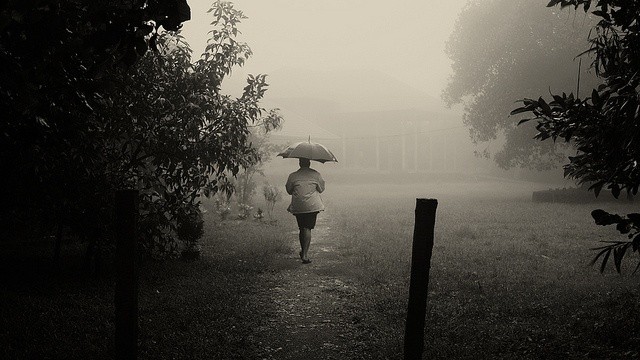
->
[299,250,303,257]
[302,258,312,264]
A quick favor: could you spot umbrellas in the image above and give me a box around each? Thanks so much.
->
[275,136,338,164]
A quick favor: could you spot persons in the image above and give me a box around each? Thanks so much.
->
[285,158,325,264]
